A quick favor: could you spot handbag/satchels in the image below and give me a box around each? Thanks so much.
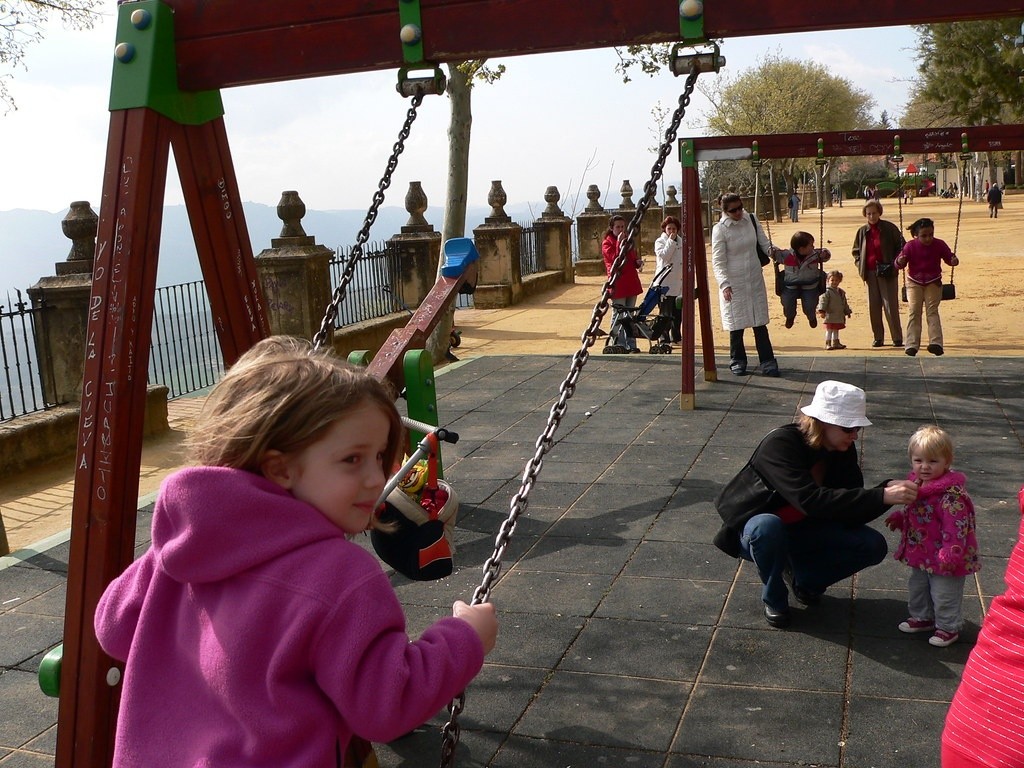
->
[371,433,458,580]
[749,213,770,267]
[875,260,896,277]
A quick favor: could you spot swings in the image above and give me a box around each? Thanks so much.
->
[894,151,968,303]
[750,160,829,300]
[302,30,733,768]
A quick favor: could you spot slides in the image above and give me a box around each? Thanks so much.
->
[887,181,906,198]
[920,178,936,197]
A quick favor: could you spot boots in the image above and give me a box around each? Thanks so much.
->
[833,339,847,349]
[825,339,833,350]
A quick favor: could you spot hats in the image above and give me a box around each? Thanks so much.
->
[800,380,874,429]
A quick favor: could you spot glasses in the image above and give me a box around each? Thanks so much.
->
[727,203,743,213]
[824,423,860,434]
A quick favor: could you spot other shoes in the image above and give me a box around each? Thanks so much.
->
[629,348,640,354]
[671,338,682,345]
[905,348,917,356]
[785,311,797,328]
[766,367,781,377]
[927,344,944,356]
[733,367,747,376]
[809,317,817,328]
[873,339,884,346]
[893,340,903,347]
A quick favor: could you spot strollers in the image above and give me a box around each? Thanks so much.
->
[603,263,674,354]
[940,190,949,198]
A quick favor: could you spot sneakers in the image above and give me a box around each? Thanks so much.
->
[899,614,935,631]
[929,629,958,646]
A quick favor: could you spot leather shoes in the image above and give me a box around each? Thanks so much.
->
[783,571,819,606]
[764,599,789,625]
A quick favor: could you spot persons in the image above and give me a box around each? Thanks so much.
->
[788,193,800,223]
[817,270,852,350]
[93,333,499,768]
[904,191,908,204]
[1000,183,1005,194]
[711,191,782,377]
[942,485,1024,768]
[653,216,683,348]
[988,183,1001,218]
[712,379,916,627]
[601,215,643,353]
[895,218,959,356]
[864,186,875,202]
[947,182,958,197]
[770,231,831,329]
[908,189,914,204]
[884,425,981,645]
[985,180,989,193]
[852,198,908,348]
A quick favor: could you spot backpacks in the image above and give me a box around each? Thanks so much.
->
[787,196,794,209]
